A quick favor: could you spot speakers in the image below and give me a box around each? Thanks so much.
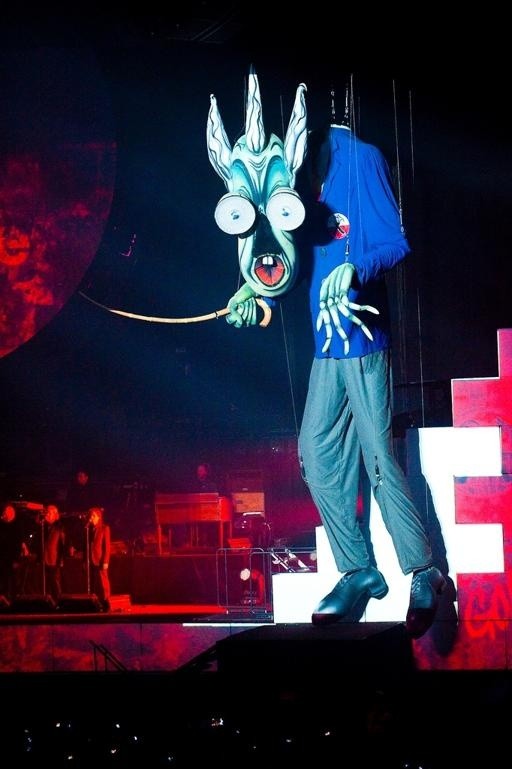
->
[62,594,104,612]
[12,595,56,613]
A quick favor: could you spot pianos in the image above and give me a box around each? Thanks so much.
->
[155,492,235,555]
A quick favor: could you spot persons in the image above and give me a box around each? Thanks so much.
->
[65,468,97,506]
[191,464,215,492]
[85,506,116,612]
[1,502,28,612]
[205,62,447,638]
[40,503,66,610]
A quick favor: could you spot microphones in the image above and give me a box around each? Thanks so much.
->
[83,518,93,528]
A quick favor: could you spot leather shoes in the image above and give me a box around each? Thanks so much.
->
[407,567,447,639]
[312,567,388,626]
